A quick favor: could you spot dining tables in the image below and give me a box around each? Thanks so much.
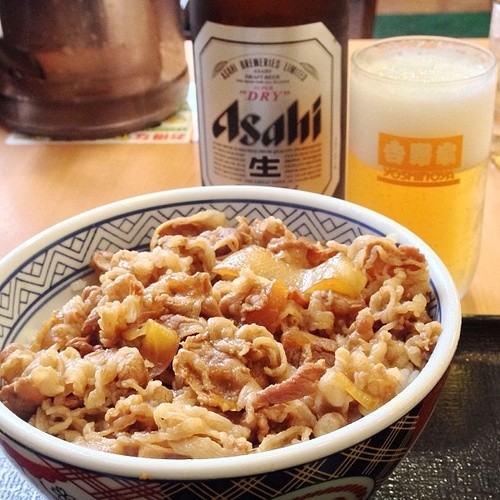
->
[0,27,500,500]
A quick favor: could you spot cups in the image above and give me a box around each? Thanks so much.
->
[348,35,498,307]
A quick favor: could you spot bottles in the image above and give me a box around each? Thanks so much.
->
[188,0,376,219]
[1,0,188,143]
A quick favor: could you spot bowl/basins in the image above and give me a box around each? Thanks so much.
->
[1,185,464,500]
